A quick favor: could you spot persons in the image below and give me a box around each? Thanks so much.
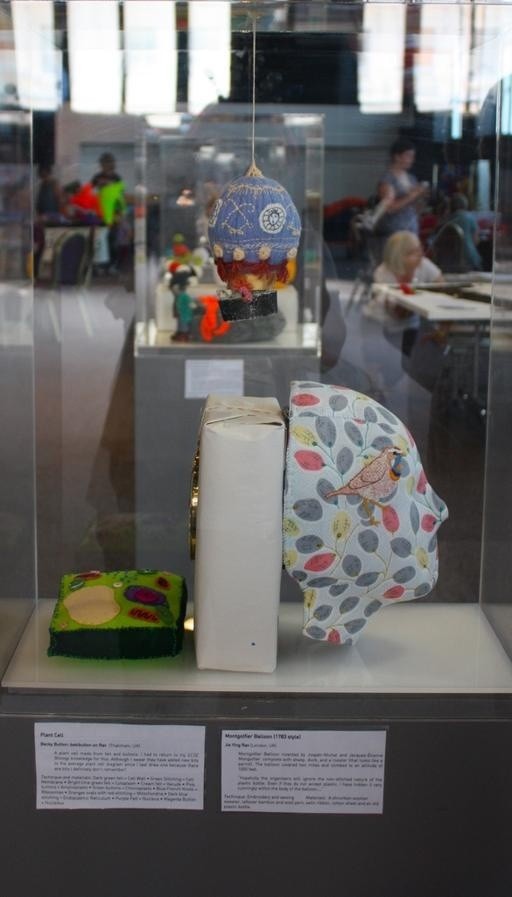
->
[360,134,489,388]
[0,151,140,293]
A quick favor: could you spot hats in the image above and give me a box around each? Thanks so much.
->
[208,167,303,265]
